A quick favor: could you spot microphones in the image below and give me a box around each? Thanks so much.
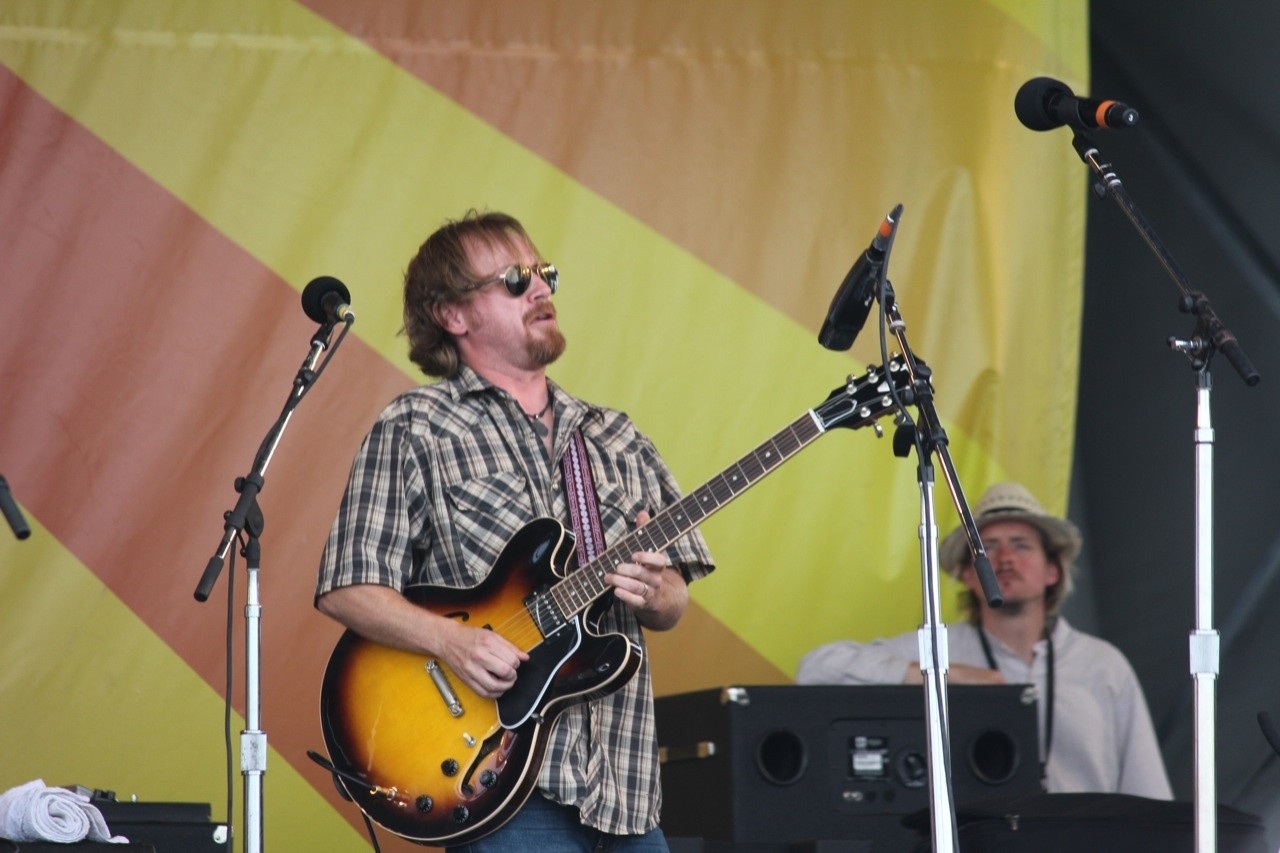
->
[1015,77,1139,132]
[301,276,355,323]
[818,204,905,352]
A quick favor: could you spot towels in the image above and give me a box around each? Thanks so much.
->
[0,778,130,844]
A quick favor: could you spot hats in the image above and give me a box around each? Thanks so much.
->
[942,484,1082,582]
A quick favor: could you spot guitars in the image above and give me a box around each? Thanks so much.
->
[318,349,925,848]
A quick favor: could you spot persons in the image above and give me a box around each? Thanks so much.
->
[314,213,715,853]
[797,483,1175,801]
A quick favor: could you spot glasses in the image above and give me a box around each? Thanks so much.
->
[441,261,558,298]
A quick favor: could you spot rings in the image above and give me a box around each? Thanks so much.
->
[641,584,648,596]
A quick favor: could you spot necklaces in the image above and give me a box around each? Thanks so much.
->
[526,390,551,437]
